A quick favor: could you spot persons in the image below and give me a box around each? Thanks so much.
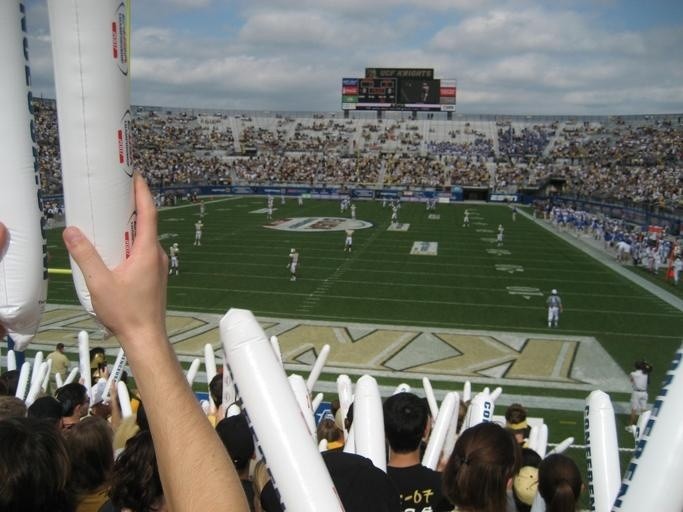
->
[496,223,505,244]
[512,118,683,285]
[0,167,248,511]
[194,220,203,246]
[285,248,300,282]
[29,100,221,218]
[343,223,355,252]
[628,359,650,433]
[221,108,512,228]
[420,81,431,104]
[166,242,180,277]
[544,289,566,326]
[1,344,583,512]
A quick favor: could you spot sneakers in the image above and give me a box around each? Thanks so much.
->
[625,426,632,433]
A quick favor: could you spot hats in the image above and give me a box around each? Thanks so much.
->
[513,448,542,507]
